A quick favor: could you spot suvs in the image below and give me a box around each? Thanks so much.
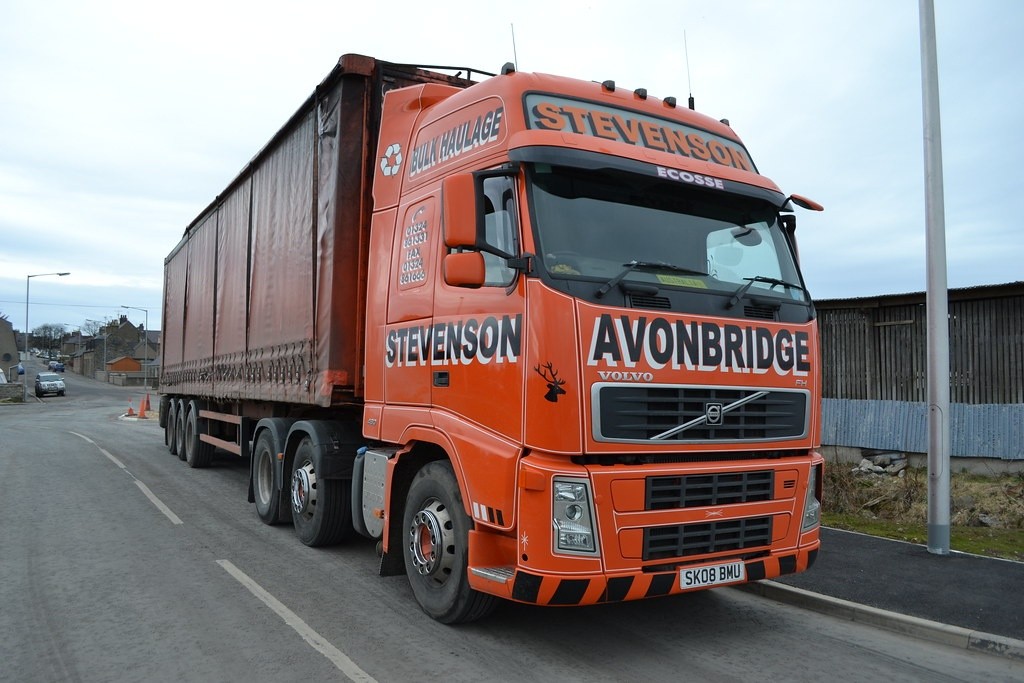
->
[35,371,66,397]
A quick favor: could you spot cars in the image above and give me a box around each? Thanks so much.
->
[48,361,65,372]
[18,363,24,375]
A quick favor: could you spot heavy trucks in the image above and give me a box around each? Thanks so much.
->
[157,53,825,625]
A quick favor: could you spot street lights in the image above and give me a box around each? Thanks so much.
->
[64,324,81,373]
[122,305,147,391]
[86,319,106,371]
[23,273,71,403]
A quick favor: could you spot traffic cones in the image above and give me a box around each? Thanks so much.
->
[143,392,153,411]
[138,397,149,419]
[124,396,137,417]
